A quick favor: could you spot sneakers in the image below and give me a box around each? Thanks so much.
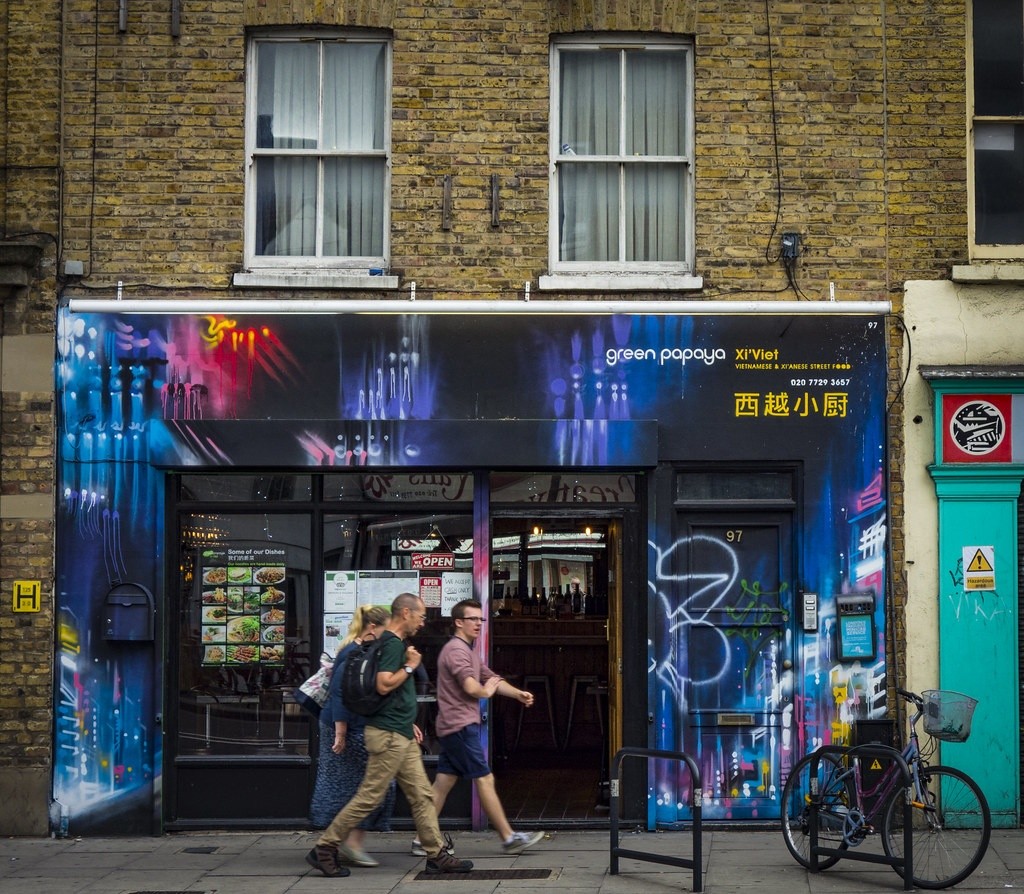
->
[305,845,351,878]
[502,831,545,854]
[411,841,455,856]
[425,832,474,874]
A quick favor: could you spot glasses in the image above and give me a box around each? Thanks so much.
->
[461,616,486,623]
[409,608,426,621]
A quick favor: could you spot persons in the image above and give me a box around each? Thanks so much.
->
[411,600,545,856]
[306,592,474,878]
[310,606,395,865]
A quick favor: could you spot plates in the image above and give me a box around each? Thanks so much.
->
[202,591,226,605]
[227,606,243,615]
[261,591,285,604]
[260,610,285,624]
[262,626,284,641]
[202,570,227,586]
[253,569,285,585]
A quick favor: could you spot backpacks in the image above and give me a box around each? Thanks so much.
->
[341,633,401,718]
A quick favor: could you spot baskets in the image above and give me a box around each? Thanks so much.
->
[921,690,979,743]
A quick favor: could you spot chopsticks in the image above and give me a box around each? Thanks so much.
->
[210,632,224,640]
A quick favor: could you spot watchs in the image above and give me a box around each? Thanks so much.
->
[402,666,413,675]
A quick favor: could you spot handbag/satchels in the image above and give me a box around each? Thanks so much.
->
[293,652,336,719]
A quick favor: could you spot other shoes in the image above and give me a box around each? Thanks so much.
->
[337,843,380,867]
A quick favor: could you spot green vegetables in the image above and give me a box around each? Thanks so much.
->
[207,587,285,663]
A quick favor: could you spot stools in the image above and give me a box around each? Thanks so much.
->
[564,673,604,751]
[513,675,557,752]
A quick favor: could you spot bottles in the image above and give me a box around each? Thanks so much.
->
[503,583,584,618]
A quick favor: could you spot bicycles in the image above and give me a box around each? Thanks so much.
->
[780,688,993,891]
